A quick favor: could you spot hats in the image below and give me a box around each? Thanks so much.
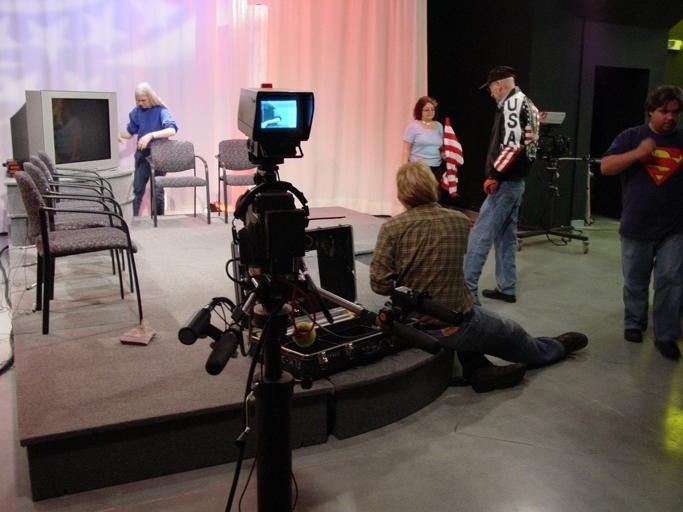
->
[478,66,515,89]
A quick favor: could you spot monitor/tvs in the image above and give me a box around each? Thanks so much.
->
[10,90,120,174]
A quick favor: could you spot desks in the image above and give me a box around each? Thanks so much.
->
[4,166,136,247]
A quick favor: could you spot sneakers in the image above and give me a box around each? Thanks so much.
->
[471,289,679,393]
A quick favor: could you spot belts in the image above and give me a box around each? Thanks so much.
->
[415,309,473,331]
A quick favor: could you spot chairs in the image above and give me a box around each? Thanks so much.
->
[217,137,280,223]
[11,150,147,335]
[146,136,211,227]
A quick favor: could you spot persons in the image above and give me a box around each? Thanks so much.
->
[113,82,180,216]
[599,84,682,362]
[399,95,443,183]
[366,159,590,395]
[458,63,539,306]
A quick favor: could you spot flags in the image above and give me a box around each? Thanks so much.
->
[441,118,469,198]
